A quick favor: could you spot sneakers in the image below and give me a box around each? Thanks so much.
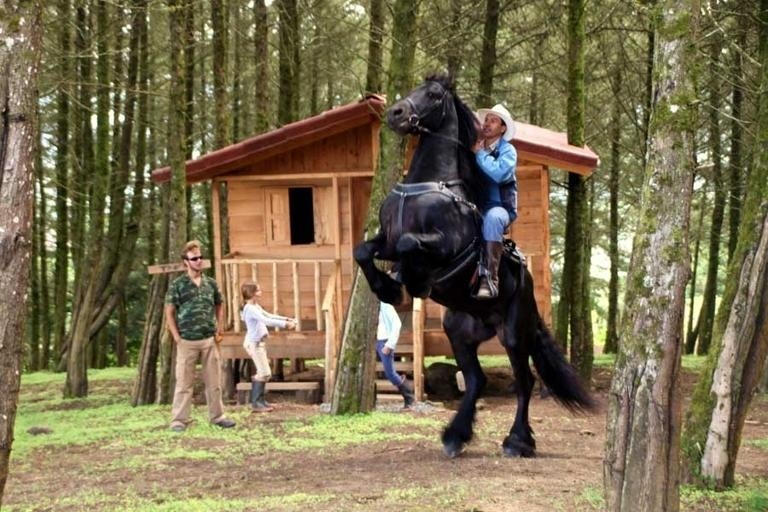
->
[215,418,235,427]
[171,425,185,432]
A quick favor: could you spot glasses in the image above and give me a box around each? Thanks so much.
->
[189,255,202,261]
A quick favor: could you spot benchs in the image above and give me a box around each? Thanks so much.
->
[237,381,320,406]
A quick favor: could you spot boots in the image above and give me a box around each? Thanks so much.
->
[397,375,416,412]
[252,380,273,412]
[476,240,503,298]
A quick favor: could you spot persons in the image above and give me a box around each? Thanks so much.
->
[468,98,520,303]
[239,281,299,412]
[373,299,414,410]
[163,240,236,430]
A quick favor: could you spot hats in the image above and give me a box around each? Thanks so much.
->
[477,104,515,141]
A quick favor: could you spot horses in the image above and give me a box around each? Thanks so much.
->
[352,72,601,458]
